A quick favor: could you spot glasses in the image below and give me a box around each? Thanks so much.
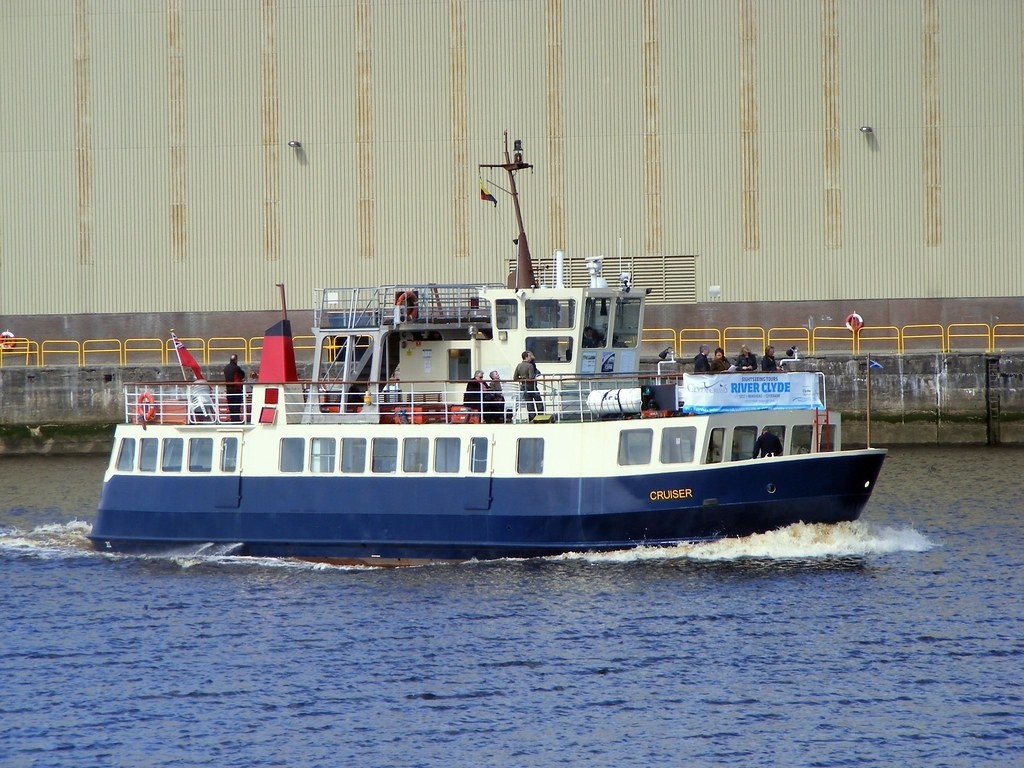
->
[479,377,483,378]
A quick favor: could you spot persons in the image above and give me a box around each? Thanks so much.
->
[513,351,544,424]
[761,345,781,373]
[582,326,603,348]
[735,343,758,373]
[752,427,783,459]
[223,354,246,424]
[463,370,505,424]
[711,347,731,373]
[693,344,712,374]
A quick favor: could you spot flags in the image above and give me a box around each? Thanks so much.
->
[171,331,214,391]
[480,182,498,208]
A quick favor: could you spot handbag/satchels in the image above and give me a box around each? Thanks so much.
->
[234,372,243,391]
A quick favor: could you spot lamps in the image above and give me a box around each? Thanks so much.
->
[860,127,873,133]
[659,347,672,359]
[288,141,302,148]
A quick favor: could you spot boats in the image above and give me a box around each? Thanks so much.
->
[84,129,890,568]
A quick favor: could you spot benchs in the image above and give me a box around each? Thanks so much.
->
[379,390,453,423]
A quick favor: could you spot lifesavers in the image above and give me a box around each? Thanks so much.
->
[397,290,419,322]
[845,313,864,333]
[138,391,155,420]
[0,332,15,350]
